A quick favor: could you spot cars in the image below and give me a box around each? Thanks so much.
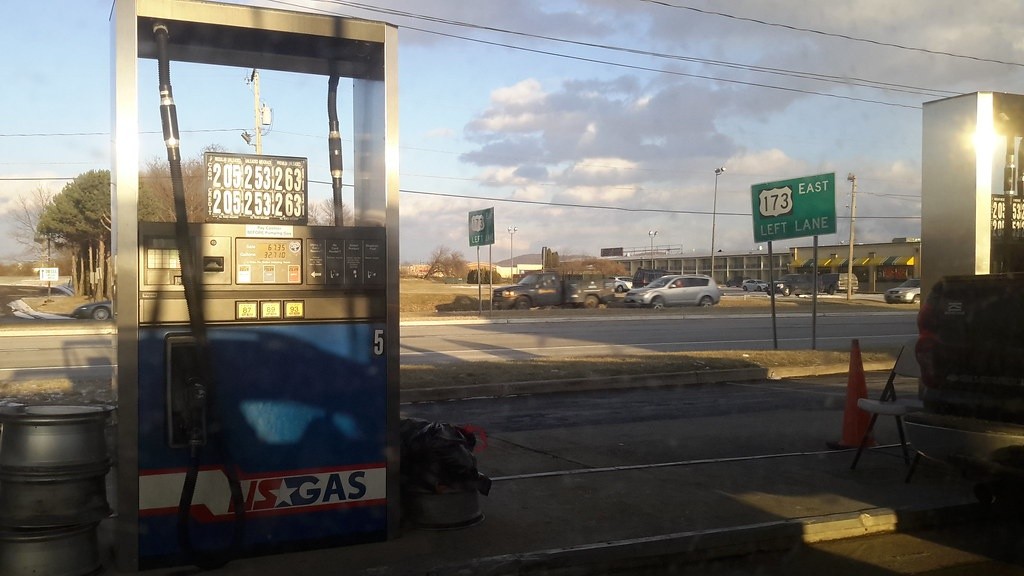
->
[74,300,111,321]
[883,278,921,305]
[726,277,751,287]
[742,278,768,291]
[608,276,634,293]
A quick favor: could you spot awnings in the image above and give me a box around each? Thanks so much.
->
[790,255,915,267]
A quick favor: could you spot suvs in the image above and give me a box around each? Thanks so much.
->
[767,274,816,297]
[820,273,859,295]
[632,269,668,288]
[623,274,720,311]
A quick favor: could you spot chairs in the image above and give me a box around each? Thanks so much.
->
[850,346,921,483]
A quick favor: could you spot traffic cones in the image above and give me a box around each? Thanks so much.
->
[824,336,882,451]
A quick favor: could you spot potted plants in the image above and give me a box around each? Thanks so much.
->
[904,411,1024,479]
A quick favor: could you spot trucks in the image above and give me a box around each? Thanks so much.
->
[493,270,616,310]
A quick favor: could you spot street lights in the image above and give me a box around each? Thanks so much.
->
[710,166,724,275]
[845,173,856,301]
[649,230,659,270]
